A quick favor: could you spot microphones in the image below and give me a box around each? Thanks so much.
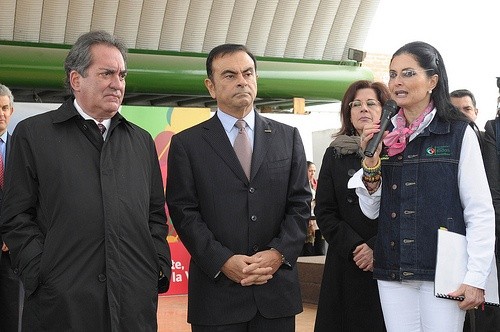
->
[363,99,397,157]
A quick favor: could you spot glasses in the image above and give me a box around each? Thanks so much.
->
[387,68,434,80]
[349,100,381,110]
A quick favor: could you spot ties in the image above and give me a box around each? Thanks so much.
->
[233,120,252,182]
[98,124,106,135]
[0,152,4,191]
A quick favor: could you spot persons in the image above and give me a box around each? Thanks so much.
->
[4,30,173,332]
[305,160,325,257]
[0,84,25,332]
[347,40,496,332]
[449,79,500,332]
[312,80,396,332]
[165,43,312,332]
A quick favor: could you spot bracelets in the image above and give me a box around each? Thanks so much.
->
[281,256,286,265]
[363,160,381,194]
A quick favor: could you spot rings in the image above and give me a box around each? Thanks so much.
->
[474,307,478,309]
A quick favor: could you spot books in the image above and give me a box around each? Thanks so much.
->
[433,228,499,307]
